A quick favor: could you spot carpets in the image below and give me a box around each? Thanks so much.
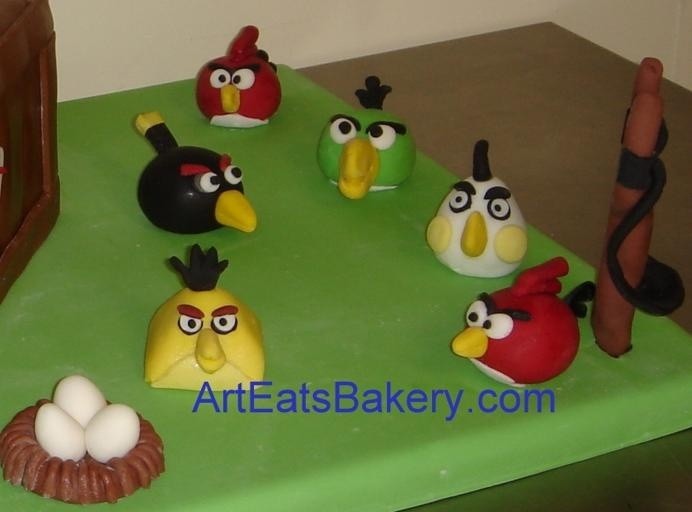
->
[0,21,692,511]
